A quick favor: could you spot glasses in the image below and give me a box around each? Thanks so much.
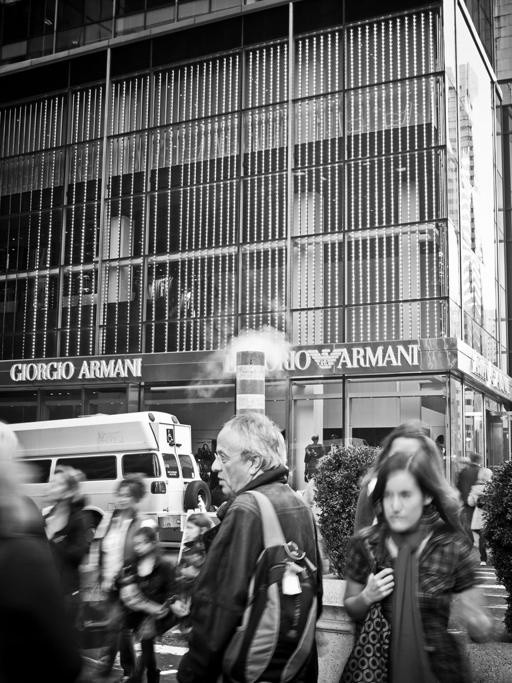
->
[214,451,245,462]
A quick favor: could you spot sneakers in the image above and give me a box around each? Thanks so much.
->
[138,673,160,683]
[119,674,135,683]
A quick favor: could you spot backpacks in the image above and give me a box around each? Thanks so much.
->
[221,491,320,683]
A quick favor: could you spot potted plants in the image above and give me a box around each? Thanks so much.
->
[310,447,379,605]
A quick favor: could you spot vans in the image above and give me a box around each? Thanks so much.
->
[1,412,213,552]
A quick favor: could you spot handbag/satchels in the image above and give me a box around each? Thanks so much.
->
[339,604,390,683]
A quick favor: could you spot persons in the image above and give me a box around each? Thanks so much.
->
[95,472,146,681]
[200,441,211,458]
[353,420,446,533]
[456,451,482,543]
[468,466,496,565]
[176,409,325,682]
[304,434,325,483]
[337,449,491,682]
[1,422,85,680]
[107,525,181,682]
[42,466,92,624]
